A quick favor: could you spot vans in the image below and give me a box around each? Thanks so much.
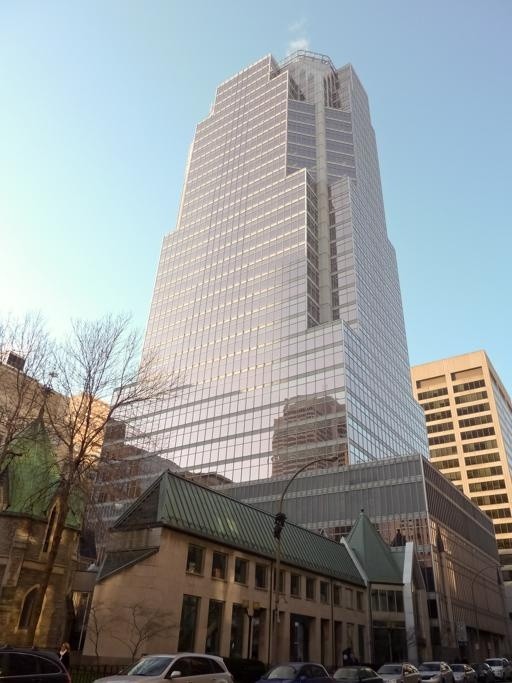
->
[1,647,75,683]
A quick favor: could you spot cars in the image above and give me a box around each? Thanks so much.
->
[92,653,234,683]
[259,656,512,682]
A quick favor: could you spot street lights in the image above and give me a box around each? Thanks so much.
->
[470,563,504,662]
[267,453,341,668]
